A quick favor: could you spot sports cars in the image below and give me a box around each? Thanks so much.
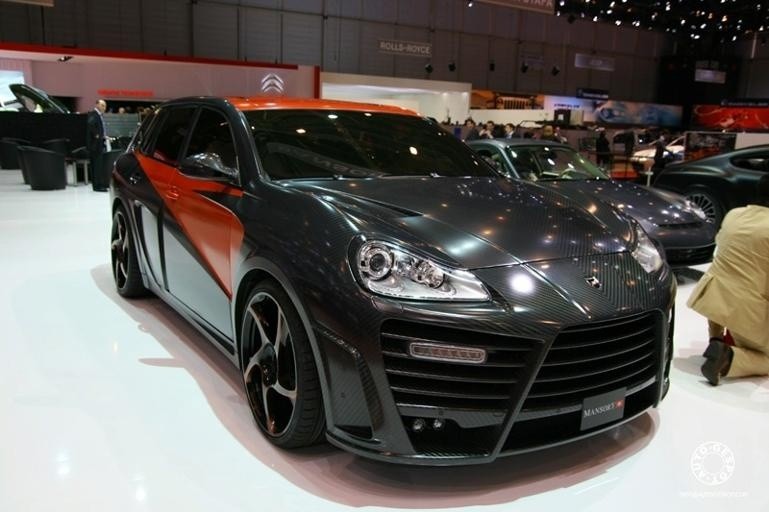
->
[0,84,88,146]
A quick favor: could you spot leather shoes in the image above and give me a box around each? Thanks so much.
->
[93,186,108,192]
[701,339,729,385]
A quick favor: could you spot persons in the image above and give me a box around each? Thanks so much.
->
[542,125,568,144]
[686,175,769,387]
[654,134,674,169]
[86,99,109,192]
[462,117,522,139]
[596,130,609,167]
[524,132,541,140]
